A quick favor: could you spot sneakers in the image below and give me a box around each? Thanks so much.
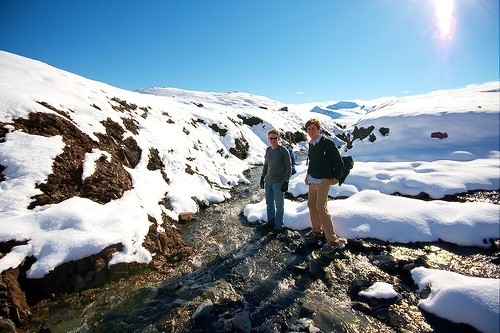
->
[320,242,346,254]
[304,230,323,240]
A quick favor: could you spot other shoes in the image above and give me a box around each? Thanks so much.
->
[265,223,274,228]
[272,229,282,236]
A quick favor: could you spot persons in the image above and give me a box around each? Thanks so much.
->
[260,129,296,232]
[305,119,344,254]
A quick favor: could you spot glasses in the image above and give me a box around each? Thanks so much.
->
[270,137,278,140]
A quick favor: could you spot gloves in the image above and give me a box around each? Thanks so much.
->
[281,180,288,192]
[260,177,265,189]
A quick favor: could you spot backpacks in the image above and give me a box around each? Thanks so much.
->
[338,155,354,186]
